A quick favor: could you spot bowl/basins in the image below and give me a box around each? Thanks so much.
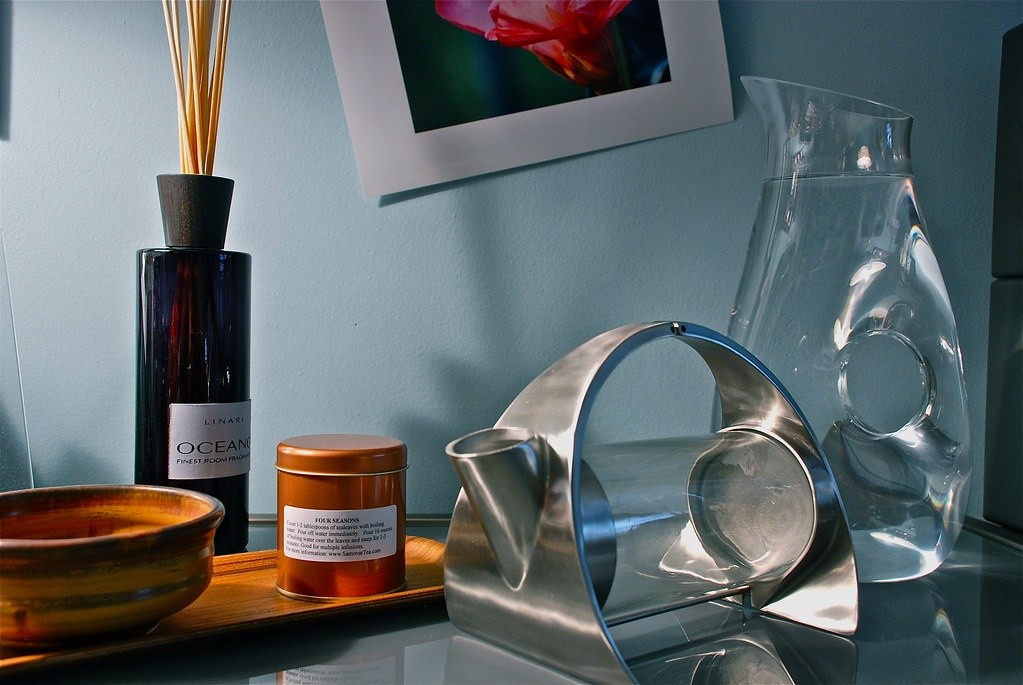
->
[0,484,225,654]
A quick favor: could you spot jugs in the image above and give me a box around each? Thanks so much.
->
[728,77,970,583]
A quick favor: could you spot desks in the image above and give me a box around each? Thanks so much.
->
[0,508,1023,685]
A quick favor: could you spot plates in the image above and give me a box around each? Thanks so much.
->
[0,534,444,668]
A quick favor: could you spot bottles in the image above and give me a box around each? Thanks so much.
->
[276,435,410,599]
